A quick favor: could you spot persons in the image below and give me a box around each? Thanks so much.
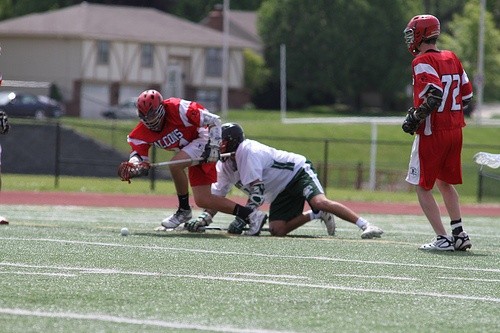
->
[0,75,11,224]
[127,90,269,236]
[185,121,384,239]
[401,14,473,250]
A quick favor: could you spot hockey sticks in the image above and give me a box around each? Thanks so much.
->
[153,223,270,232]
[117,150,236,181]
[473,152,500,169]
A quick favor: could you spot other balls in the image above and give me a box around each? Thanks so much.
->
[121,228,128,235]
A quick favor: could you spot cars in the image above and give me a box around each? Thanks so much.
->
[0,92,65,122]
[99,97,140,120]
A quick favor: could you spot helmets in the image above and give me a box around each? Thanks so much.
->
[137,90,165,130]
[221,123,243,162]
[404,15,440,56]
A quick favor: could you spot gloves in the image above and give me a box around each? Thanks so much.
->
[402,107,420,135]
[228,216,246,234]
[201,145,220,163]
[140,162,153,177]
[184,212,212,232]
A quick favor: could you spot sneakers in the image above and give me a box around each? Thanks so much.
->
[419,235,455,252]
[162,208,191,229]
[361,226,383,239]
[246,210,267,235]
[451,232,472,251]
[315,211,336,235]
